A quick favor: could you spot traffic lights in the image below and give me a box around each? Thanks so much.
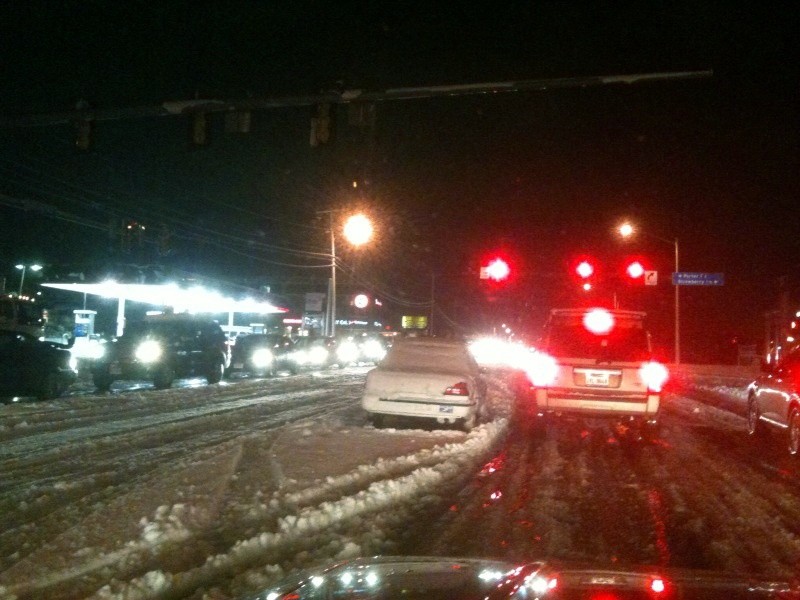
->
[627,255,644,287]
[489,256,504,288]
[576,257,596,294]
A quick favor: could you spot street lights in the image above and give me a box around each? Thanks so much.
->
[13,263,44,299]
[620,223,681,366]
[330,212,372,338]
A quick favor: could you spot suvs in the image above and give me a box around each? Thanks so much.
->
[92,312,230,391]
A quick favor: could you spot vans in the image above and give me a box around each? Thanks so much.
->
[526,307,663,427]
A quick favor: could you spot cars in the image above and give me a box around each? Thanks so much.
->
[230,321,400,378]
[743,345,800,458]
[361,335,491,434]
[0,331,76,406]
